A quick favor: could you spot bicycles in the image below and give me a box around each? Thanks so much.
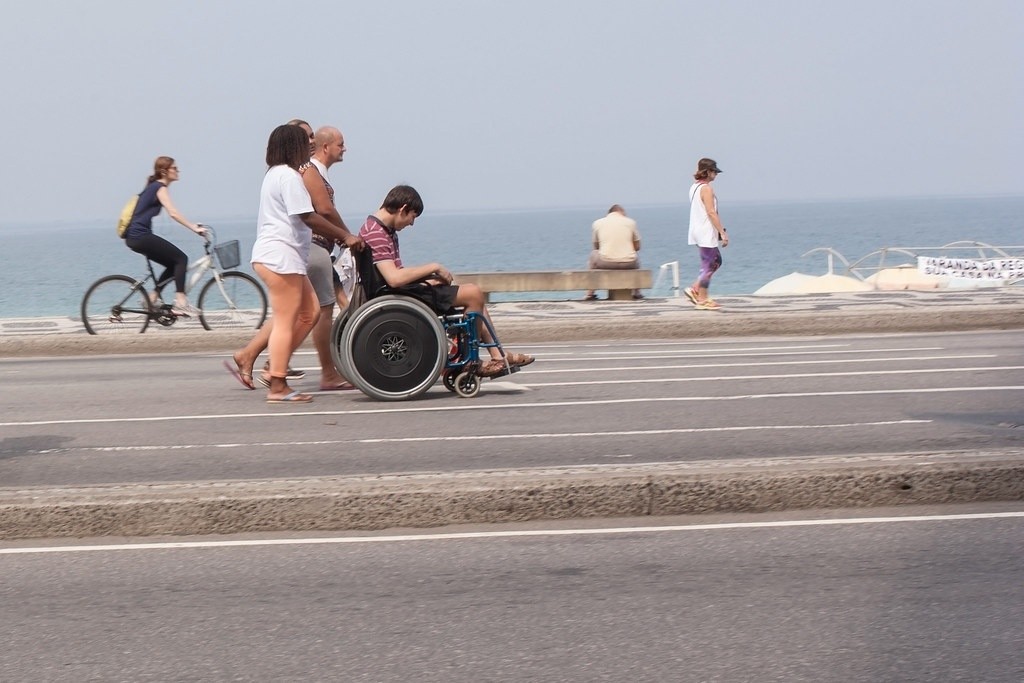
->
[81,223,268,335]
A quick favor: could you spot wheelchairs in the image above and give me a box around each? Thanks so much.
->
[330,242,521,402]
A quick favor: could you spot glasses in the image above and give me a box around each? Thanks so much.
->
[167,166,177,170]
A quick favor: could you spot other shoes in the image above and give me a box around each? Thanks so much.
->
[584,294,597,301]
[264,358,306,379]
[633,295,645,299]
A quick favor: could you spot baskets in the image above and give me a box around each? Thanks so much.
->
[214,240,240,266]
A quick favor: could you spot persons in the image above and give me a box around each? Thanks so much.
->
[682,157,730,310]
[223,118,365,405]
[584,204,646,300]
[125,156,209,318]
[355,184,535,377]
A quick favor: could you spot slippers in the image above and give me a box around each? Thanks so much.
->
[257,376,271,388]
[223,360,255,390]
[267,391,314,403]
[320,378,357,391]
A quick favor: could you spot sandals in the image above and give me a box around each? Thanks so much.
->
[464,359,505,376]
[695,300,721,310]
[491,352,535,366]
[684,286,699,305]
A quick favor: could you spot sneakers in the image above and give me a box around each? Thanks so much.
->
[171,299,202,317]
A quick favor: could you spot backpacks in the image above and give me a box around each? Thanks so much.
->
[117,195,140,239]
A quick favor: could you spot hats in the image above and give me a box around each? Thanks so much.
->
[699,162,722,173]
